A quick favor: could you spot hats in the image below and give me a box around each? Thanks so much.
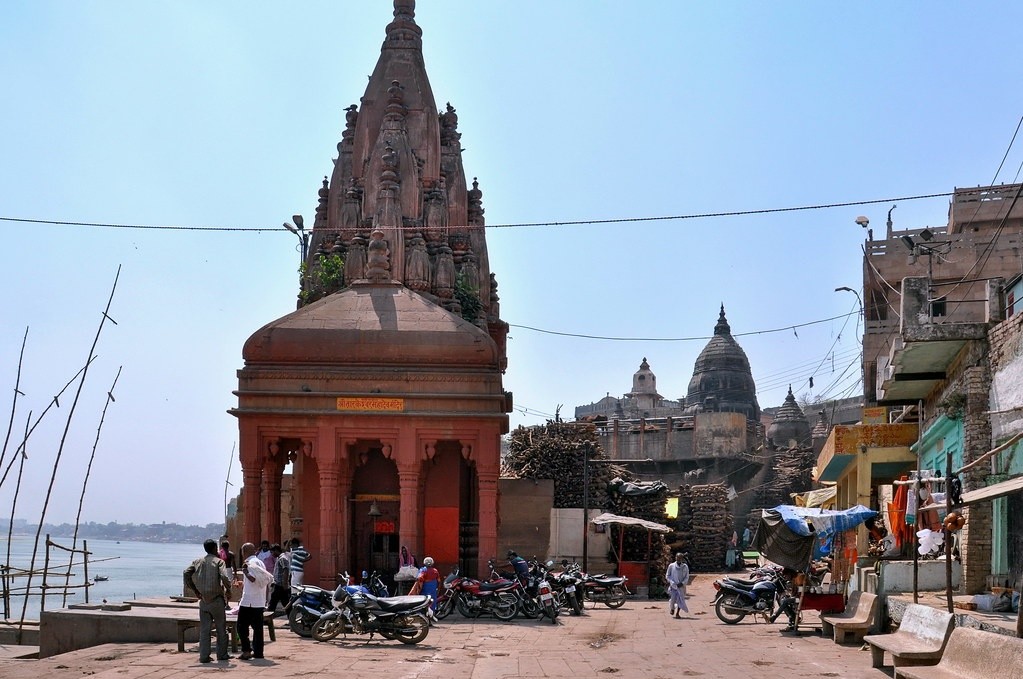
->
[506,550,513,559]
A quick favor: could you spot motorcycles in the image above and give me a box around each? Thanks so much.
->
[708,562,804,625]
[282,565,407,640]
[487,553,632,625]
[429,558,521,623]
[311,573,440,646]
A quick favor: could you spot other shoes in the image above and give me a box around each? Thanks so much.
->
[237,651,251,660]
[216,654,234,660]
[224,605,232,609]
[200,657,213,663]
[675,613,681,618]
[670,609,674,614]
[251,654,263,658]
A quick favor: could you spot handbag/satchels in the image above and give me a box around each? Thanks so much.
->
[247,564,274,590]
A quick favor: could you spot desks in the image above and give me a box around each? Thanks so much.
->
[173,613,277,654]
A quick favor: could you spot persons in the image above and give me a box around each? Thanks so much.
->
[183,538,235,662]
[236,543,267,659]
[256,538,312,619]
[218,542,234,610]
[417,557,441,621]
[560,559,570,569]
[666,553,689,618]
[399,546,414,568]
[742,525,749,551]
[762,567,813,631]
[493,550,528,582]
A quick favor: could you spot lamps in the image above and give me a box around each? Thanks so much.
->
[919,228,936,242]
[900,235,914,251]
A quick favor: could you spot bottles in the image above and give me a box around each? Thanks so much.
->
[916,529,944,555]
[810,581,844,594]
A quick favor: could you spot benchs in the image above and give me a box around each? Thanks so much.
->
[894,626,1023,679]
[819,591,878,644]
[863,603,955,676]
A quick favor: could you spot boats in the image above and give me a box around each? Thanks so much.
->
[94,576,109,581]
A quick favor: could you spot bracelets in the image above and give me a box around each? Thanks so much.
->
[256,552,259,555]
